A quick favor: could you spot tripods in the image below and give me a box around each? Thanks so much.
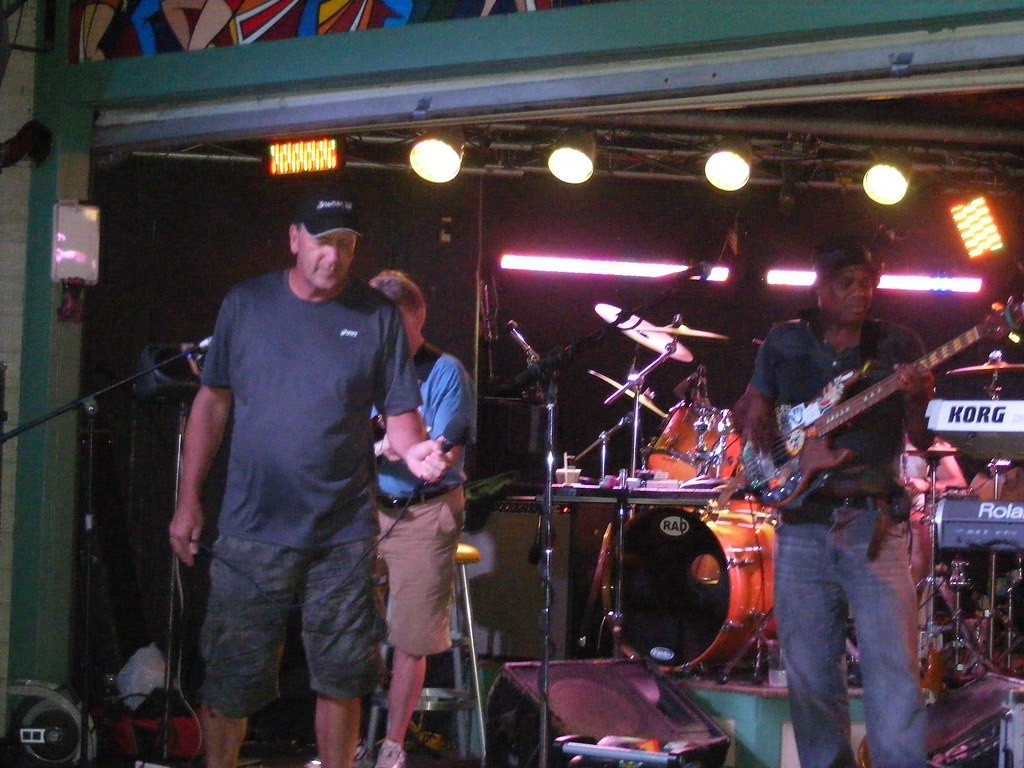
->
[915,449,1024,689]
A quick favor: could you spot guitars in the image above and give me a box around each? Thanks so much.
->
[464,471,523,500]
[742,296,1022,510]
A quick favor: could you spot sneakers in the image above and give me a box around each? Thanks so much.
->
[307,741,367,768]
[375,740,406,768]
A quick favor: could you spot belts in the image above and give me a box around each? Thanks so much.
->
[808,492,891,512]
[377,483,457,511]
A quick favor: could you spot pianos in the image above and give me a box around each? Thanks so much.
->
[933,494,1023,675]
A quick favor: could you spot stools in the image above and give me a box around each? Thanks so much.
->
[368,543,487,768]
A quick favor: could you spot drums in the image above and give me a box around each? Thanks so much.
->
[600,504,779,674]
[646,401,743,486]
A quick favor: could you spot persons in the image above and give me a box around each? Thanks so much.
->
[366,281,474,768]
[731,241,936,768]
[169,185,453,768]
[898,435,966,592]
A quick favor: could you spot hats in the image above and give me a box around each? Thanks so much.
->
[287,179,365,237]
[810,238,881,288]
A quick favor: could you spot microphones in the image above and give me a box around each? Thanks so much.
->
[200,336,214,352]
[655,262,712,284]
[529,514,543,565]
[696,366,707,399]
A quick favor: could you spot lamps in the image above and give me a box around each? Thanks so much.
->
[548,127,596,183]
[861,149,913,206]
[705,136,753,191]
[409,125,465,183]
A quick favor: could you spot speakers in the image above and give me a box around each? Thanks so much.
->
[857,672,1024,768]
[484,655,732,768]
[460,501,576,663]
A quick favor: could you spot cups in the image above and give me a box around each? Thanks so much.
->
[555,469,582,483]
[769,641,788,687]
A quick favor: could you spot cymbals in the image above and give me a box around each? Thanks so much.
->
[905,446,961,458]
[594,303,694,363]
[947,362,1023,377]
[635,324,730,342]
